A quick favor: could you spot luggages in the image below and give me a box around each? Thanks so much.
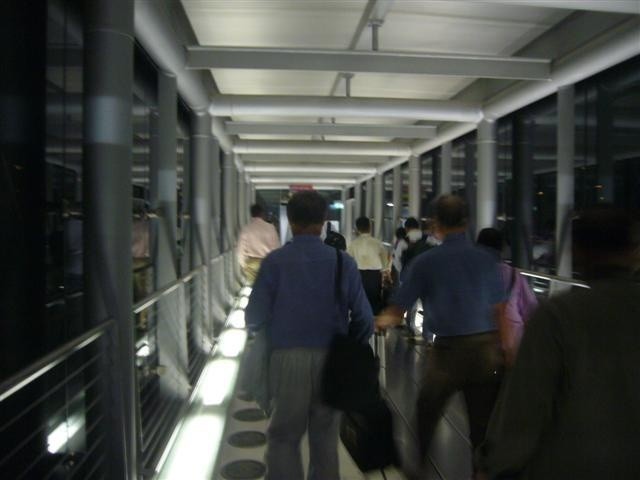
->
[340,331,397,474]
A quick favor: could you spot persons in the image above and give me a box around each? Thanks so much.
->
[322,216,440,343]
[477,227,537,349]
[376,201,509,478]
[246,190,374,478]
[236,203,280,288]
[474,203,639,478]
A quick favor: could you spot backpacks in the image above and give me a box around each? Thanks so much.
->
[402,234,434,268]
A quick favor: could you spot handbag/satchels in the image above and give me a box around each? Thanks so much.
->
[324,231,347,250]
[320,329,378,409]
